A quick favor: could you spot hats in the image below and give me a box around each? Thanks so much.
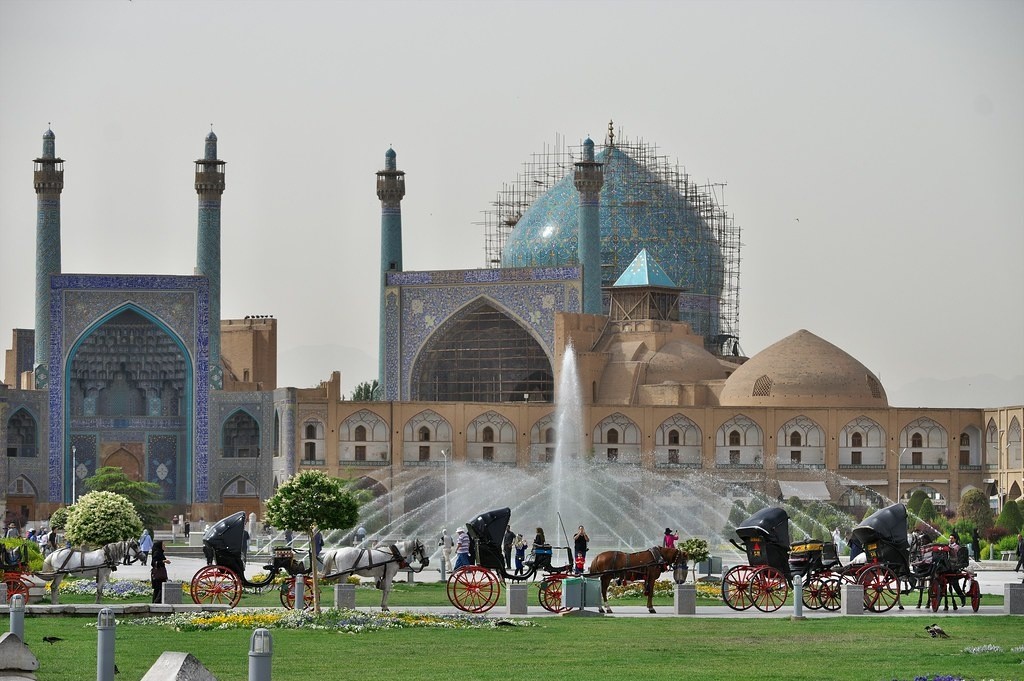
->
[664,528,672,534]
[456,527,464,532]
[40,526,45,529]
[8,523,16,527]
[28,528,34,532]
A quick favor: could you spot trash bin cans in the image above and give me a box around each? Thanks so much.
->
[584,578,602,607]
[699,558,710,573]
[561,579,582,607]
[711,557,722,574]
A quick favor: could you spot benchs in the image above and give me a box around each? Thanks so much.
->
[790,539,837,568]
[522,543,572,572]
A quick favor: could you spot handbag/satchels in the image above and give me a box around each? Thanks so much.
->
[517,555,525,561]
[151,561,168,581]
[321,539,324,546]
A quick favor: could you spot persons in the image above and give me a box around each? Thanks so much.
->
[802,527,849,555]
[202,544,214,566]
[972,528,981,562]
[908,527,961,558]
[575,551,585,575]
[151,540,171,604]
[356,523,367,542]
[1013,534,1024,572]
[515,534,528,576]
[531,528,545,555]
[241,530,250,566]
[436,527,479,574]
[503,525,516,569]
[0,523,62,558]
[935,534,966,608]
[308,526,323,574]
[263,519,271,533]
[573,526,590,575]
[663,528,679,571]
[285,528,293,547]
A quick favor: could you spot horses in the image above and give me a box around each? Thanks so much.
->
[847,532,924,610]
[43,536,147,604]
[591,546,689,614]
[323,538,430,611]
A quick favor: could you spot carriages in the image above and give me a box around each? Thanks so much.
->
[188,510,430,615]
[446,507,693,614]
[720,504,984,616]
[0,535,146,606]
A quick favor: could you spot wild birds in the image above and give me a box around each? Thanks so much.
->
[931,624,950,638]
[43,636,63,646]
[924,626,937,639]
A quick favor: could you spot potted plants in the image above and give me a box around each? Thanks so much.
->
[999,536,1017,561]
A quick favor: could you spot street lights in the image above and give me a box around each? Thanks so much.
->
[440,447,451,524]
[73,445,78,507]
[993,444,1013,515]
[889,447,908,505]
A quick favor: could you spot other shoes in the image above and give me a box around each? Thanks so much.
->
[1013,569,1018,573]
[437,568,441,573]
[506,567,511,569]
[976,560,981,562]
[669,569,673,571]
[141,563,144,565]
[447,571,453,574]
[961,597,965,607]
[144,564,147,566]
